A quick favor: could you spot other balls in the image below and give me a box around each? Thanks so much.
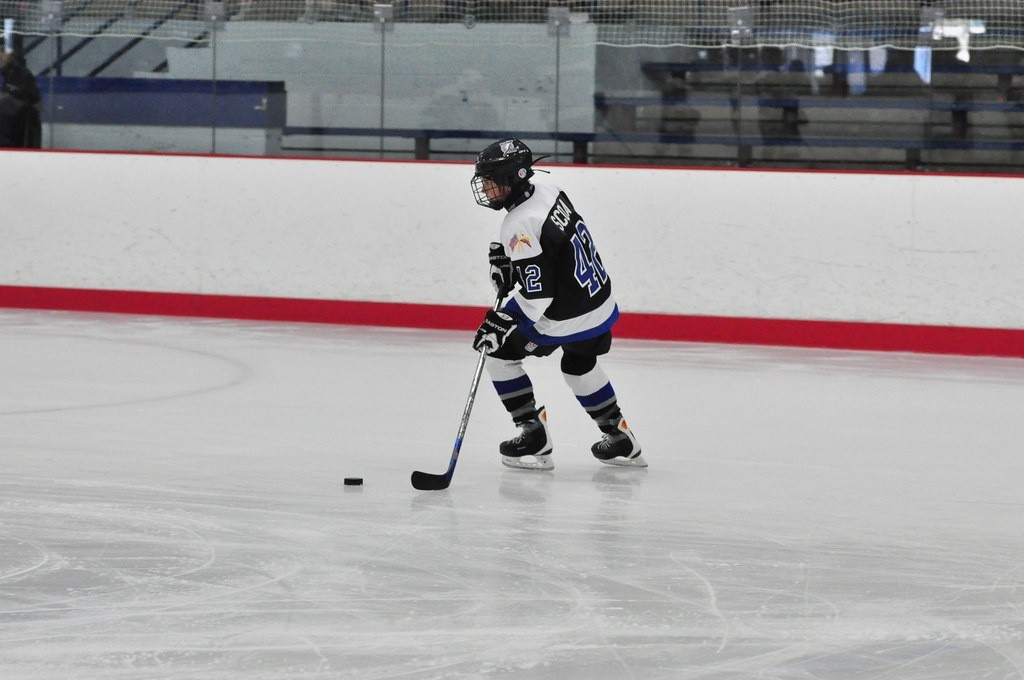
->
[344,477,363,485]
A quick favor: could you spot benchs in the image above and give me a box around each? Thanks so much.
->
[0,1,1024,177]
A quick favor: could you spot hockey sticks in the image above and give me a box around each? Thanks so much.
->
[410,290,503,492]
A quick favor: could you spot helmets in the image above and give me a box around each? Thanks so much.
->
[475,139,536,174]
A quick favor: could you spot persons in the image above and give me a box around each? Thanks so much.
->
[472,137,642,461]
[0,31,43,149]
[757,47,810,142]
[653,70,700,144]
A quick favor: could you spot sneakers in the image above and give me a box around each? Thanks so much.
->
[499,407,555,473]
[590,416,651,469]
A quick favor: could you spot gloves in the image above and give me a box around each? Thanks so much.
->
[472,309,519,354]
[487,249,517,297]
